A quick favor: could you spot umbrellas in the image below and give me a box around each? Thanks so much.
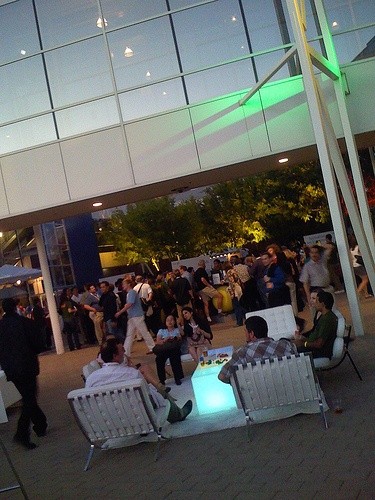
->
[0,287,28,300]
[0,264,42,286]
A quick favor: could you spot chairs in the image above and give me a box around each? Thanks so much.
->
[164,326,209,376]
[245,304,298,341]
[66,377,173,472]
[226,351,330,444]
[80,359,168,409]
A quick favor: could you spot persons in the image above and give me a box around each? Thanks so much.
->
[218,316,298,385]
[114,278,155,358]
[155,315,185,385]
[299,243,338,322]
[0,234,375,451]
[177,306,214,368]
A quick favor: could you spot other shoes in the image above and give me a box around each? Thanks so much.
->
[218,311,228,317]
[235,324,239,327]
[14,433,36,449]
[208,320,214,325]
[175,379,181,385]
[182,399,192,420]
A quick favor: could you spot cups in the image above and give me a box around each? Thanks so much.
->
[203,350,208,357]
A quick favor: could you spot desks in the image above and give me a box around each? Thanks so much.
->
[0,369,29,500]
[190,345,238,415]
[212,285,234,315]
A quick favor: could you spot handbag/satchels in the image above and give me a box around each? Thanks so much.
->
[140,297,150,312]
[353,255,364,265]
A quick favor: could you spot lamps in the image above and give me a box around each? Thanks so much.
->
[124,46,133,58]
[332,20,338,27]
[145,71,151,77]
[96,16,108,29]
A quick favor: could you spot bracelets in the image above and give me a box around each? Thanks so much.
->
[304,341,308,348]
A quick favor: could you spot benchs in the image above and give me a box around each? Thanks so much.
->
[312,309,363,382]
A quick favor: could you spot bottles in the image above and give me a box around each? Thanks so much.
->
[199,356,204,367]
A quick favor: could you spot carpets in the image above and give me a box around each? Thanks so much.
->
[98,374,330,450]
